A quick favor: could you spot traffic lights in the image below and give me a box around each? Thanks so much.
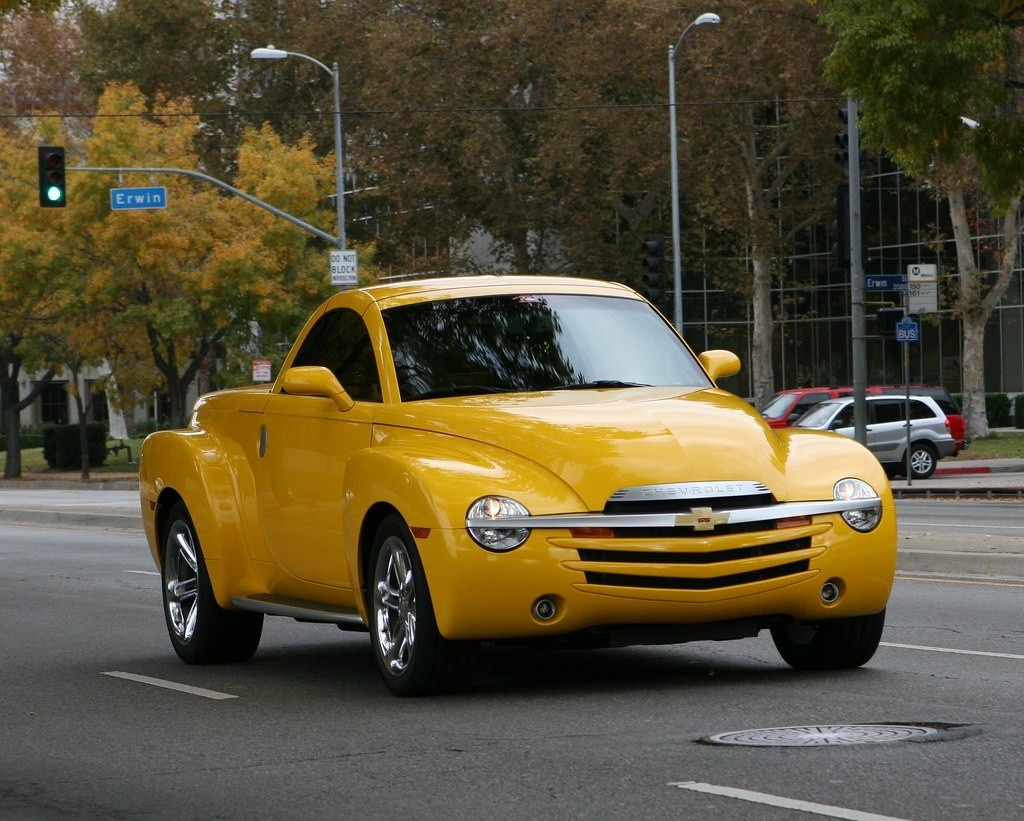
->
[38,145,65,209]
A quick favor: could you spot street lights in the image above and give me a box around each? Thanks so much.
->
[250,44,346,295]
[668,12,722,344]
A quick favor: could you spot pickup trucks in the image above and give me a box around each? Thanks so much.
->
[758,384,966,465]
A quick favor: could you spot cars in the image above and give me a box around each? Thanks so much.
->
[137,273,897,698]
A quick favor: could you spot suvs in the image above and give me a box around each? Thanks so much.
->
[786,393,956,480]
